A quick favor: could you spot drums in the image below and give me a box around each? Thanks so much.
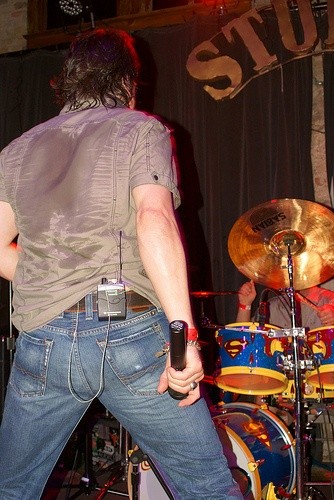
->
[196,322,224,385]
[269,325,334,401]
[212,320,289,396]
[126,401,298,500]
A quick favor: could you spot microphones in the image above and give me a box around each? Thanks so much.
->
[258,292,267,325]
[167,320,189,400]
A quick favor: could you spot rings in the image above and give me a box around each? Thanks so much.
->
[189,382,197,392]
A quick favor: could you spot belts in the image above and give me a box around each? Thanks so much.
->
[63,289,156,312]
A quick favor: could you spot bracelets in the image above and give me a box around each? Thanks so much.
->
[186,329,201,352]
[239,304,252,311]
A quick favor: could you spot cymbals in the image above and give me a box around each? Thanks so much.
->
[189,290,230,297]
[226,197,334,293]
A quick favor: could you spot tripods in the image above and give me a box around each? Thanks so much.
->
[45,419,130,500]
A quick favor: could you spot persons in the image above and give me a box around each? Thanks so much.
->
[1,28,246,500]
[236,277,334,416]
